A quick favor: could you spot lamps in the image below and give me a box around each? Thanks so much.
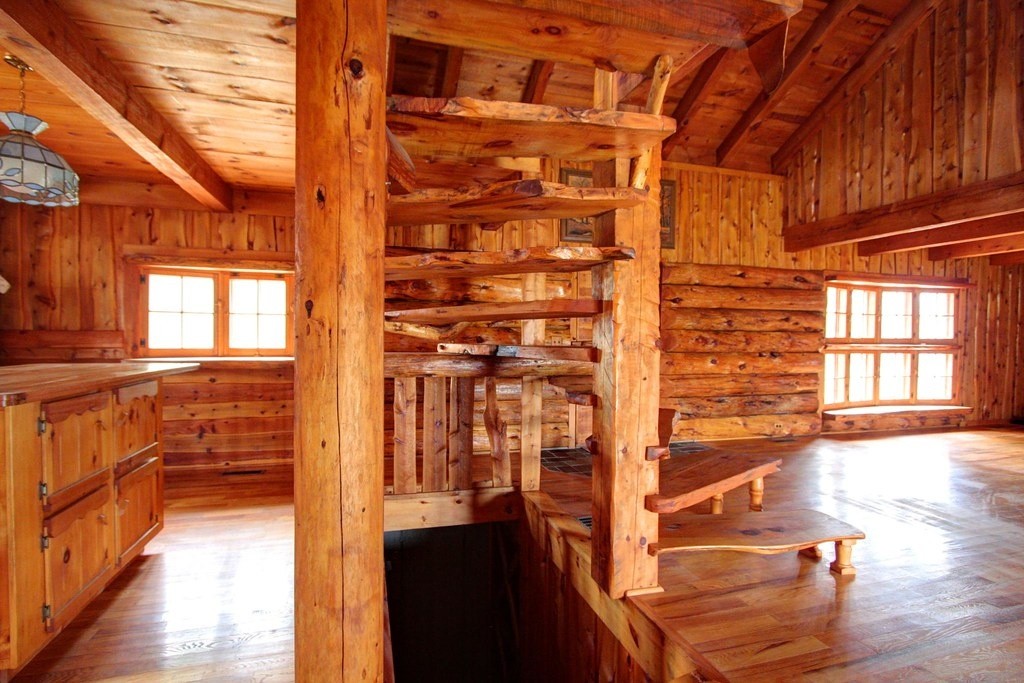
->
[0,53,80,207]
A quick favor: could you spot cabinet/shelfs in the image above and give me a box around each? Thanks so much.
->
[0,379,164,683]
